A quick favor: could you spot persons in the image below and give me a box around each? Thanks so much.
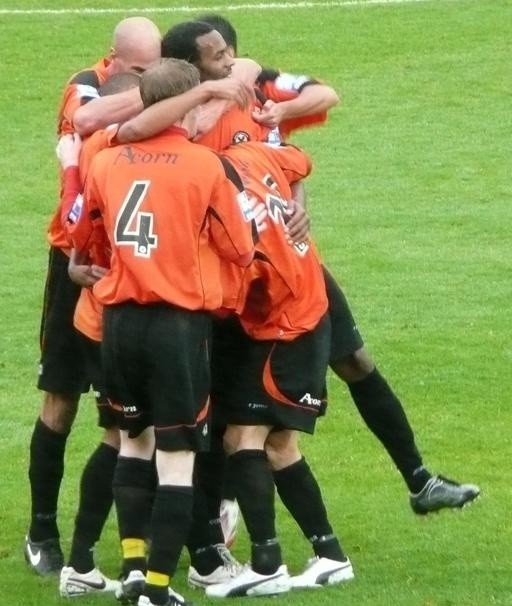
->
[23,15,481,605]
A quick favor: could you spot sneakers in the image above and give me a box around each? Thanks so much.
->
[23,530,64,577]
[59,564,122,596]
[204,560,290,596]
[289,555,356,589]
[219,497,241,548]
[187,557,249,587]
[410,474,481,517]
[115,569,192,606]
